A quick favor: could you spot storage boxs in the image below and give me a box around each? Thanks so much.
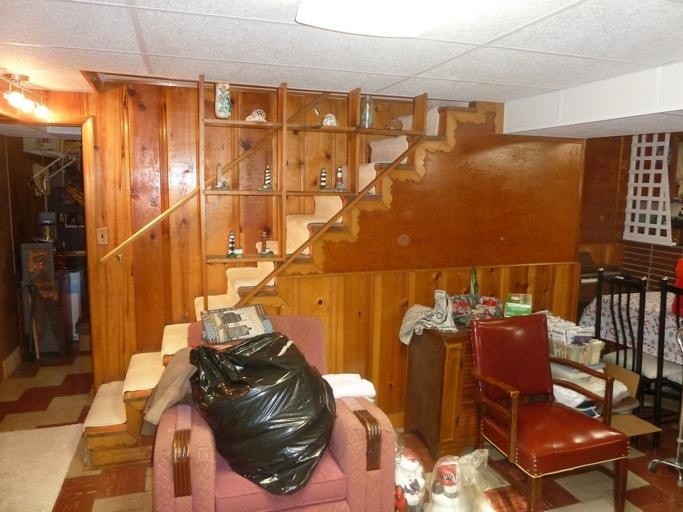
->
[606,362,663,437]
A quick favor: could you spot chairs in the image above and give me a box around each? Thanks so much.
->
[152,316,398,512]
[653,277,683,448]
[594,268,683,443]
[470,314,630,512]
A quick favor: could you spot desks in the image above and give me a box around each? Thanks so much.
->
[579,292,682,366]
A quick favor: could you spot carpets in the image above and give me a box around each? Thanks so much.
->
[0,422,84,512]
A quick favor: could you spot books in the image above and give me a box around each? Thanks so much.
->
[541,317,595,361]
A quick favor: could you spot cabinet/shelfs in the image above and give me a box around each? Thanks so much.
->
[403,323,480,461]
[198,75,428,296]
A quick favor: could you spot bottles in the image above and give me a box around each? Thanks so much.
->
[40,220,56,241]
[361,95,375,128]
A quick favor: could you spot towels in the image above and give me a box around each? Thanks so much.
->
[399,290,458,345]
[321,373,376,402]
